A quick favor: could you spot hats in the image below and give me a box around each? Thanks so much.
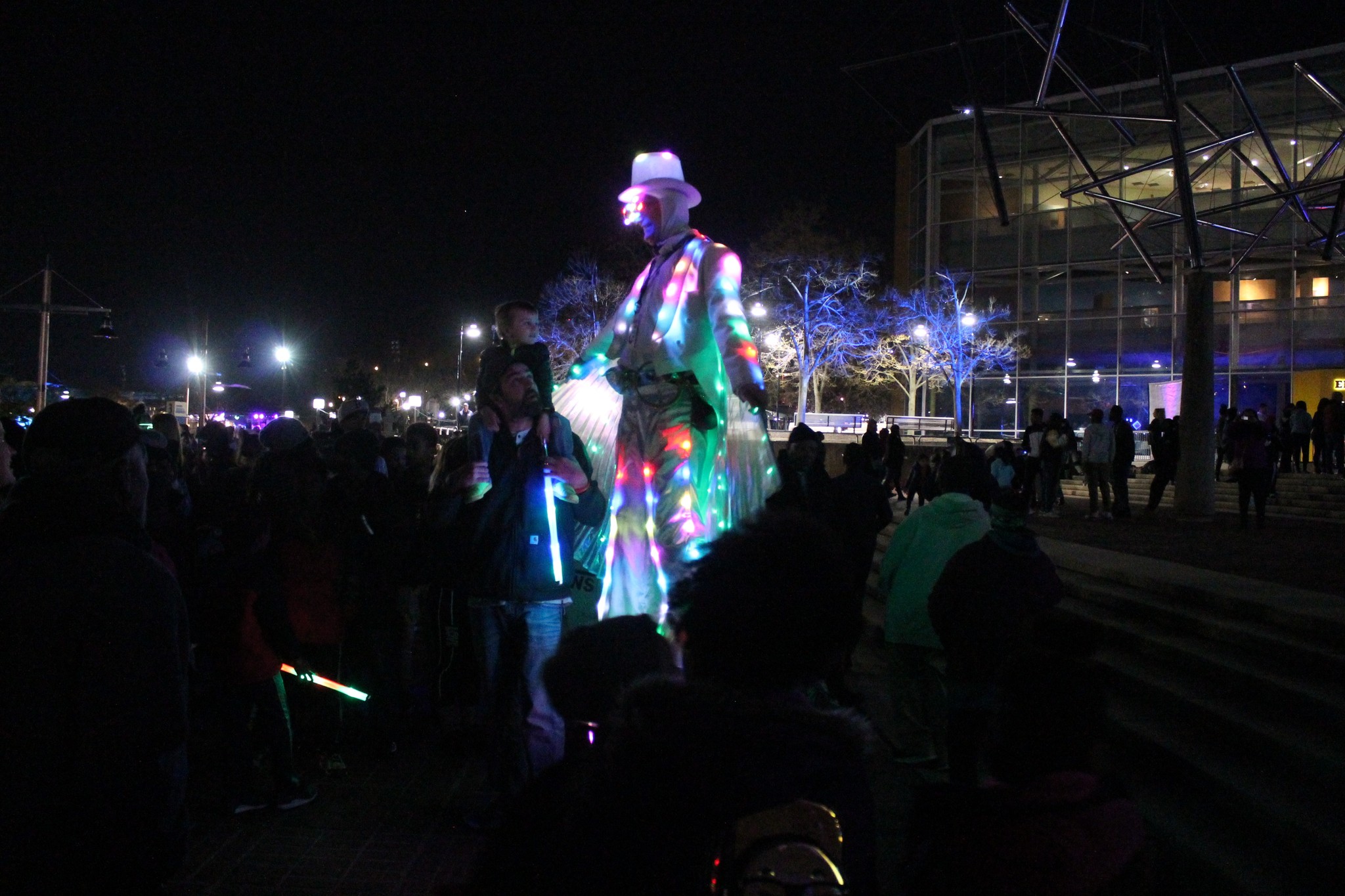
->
[24,394,168,463]
[618,152,702,210]
[337,399,370,422]
[259,417,311,451]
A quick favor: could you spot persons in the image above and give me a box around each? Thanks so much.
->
[460,296,583,510]
[555,147,770,615]
[0,357,1345,896]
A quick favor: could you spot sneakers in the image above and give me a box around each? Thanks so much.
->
[551,482,580,506]
[463,481,492,504]
